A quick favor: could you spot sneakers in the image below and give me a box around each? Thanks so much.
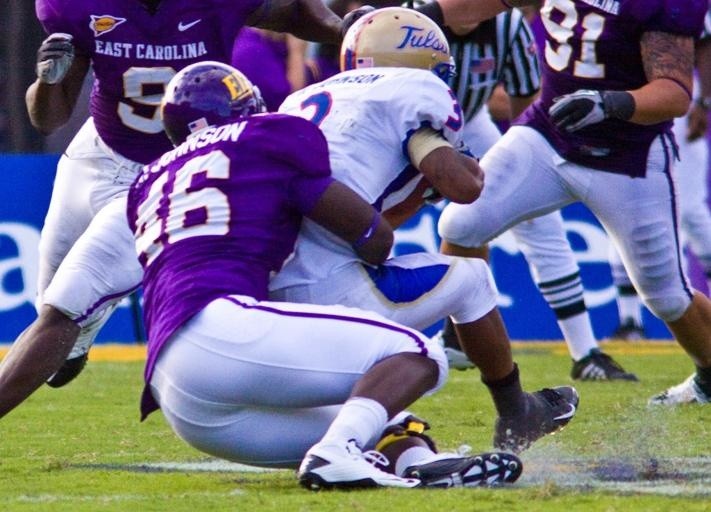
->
[432,329,472,371]
[494,386,579,454]
[571,346,634,381]
[46,302,115,387]
[299,443,422,489]
[403,452,522,487]
[649,376,705,405]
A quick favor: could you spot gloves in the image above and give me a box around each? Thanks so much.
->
[37,32,73,84]
[549,90,610,133]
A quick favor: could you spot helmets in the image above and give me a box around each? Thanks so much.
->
[341,7,456,85]
[161,62,264,147]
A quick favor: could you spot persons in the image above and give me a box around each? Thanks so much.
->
[0,0,710,452]
[127,60,524,494]
